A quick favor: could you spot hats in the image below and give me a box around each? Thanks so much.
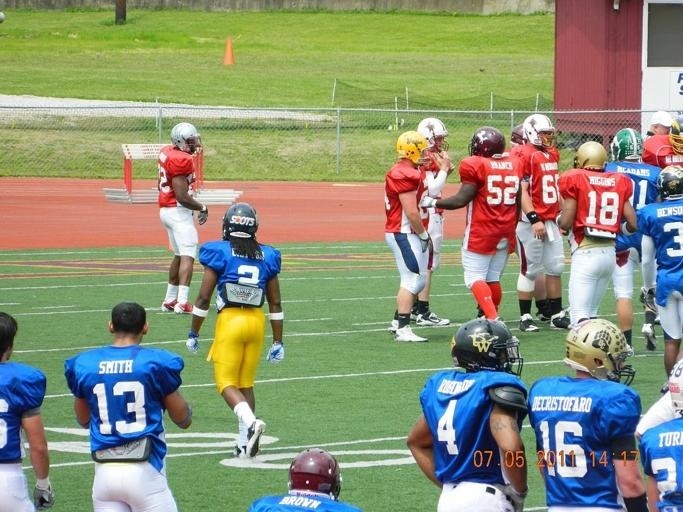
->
[649,111,672,128]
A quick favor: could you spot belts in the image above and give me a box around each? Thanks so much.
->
[224,303,261,309]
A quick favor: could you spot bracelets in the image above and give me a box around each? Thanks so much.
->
[202,204,206,212]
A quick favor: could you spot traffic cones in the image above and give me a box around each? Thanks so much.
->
[221,38,238,66]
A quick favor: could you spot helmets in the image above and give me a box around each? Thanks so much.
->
[468,126,507,156]
[668,358,683,420]
[565,318,636,388]
[395,130,431,167]
[170,122,198,154]
[416,117,449,148]
[222,202,258,240]
[574,141,609,171]
[287,447,342,499]
[669,116,683,157]
[450,318,523,376]
[611,128,645,162]
[510,113,556,146]
[657,166,683,201]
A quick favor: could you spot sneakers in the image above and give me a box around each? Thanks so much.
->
[641,323,658,351]
[387,318,429,343]
[410,308,450,326]
[160,300,188,312]
[246,418,267,458]
[519,319,541,333]
[174,301,200,314]
[535,309,571,330]
[230,445,249,459]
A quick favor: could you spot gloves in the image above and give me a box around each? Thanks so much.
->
[186,330,200,354]
[32,477,56,509]
[266,341,286,364]
[502,482,529,511]
[197,206,209,226]
[639,286,658,314]
[419,195,438,209]
[418,231,434,255]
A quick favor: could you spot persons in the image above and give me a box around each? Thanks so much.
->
[184,200,287,459]
[524,318,647,511]
[406,319,531,511]
[0,310,55,512]
[156,121,209,316]
[62,300,193,512]
[247,447,365,512]
[637,358,682,511]
